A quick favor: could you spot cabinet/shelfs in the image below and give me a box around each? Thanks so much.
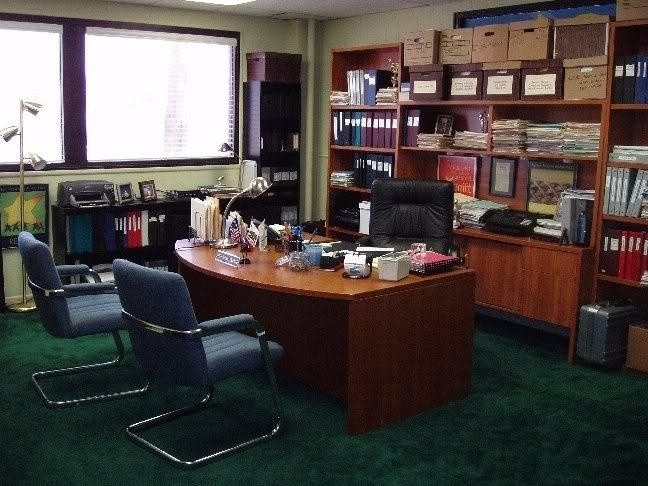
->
[172,189,257,263]
[398,99,602,371]
[326,41,401,247]
[590,19,647,284]
[48,197,173,284]
[242,77,302,227]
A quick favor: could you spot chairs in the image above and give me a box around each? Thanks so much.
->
[368,178,454,263]
[112,259,289,469]
[17,231,150,408]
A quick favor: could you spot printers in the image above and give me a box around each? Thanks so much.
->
[57,180,115,209]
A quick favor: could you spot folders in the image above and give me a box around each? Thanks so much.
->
[114,208,167,250]
[333,69,431,189]
[599,55,648,282]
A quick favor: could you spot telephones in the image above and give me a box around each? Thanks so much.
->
[253,219,282,244]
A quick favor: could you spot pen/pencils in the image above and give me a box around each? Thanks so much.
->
[282,221,303,240]
[308,228,317,243]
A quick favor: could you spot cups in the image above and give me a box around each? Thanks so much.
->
[307,244,323,267]
[412,242,428,259]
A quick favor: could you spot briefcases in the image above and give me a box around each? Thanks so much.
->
[576,296,641,368]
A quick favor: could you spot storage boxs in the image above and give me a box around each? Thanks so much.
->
[624,323,648,374]
[245,51,302,78]
[610,0,647,21]
[404,18,607,103]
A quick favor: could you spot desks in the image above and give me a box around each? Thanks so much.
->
[170,227,478,439]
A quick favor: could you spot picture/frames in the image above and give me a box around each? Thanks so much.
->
[138,180,157,202]
[435,115,456,135]
[115,182,135,205]
[435,154,481,197]
[451,0,616,31]
[487,154,519,199]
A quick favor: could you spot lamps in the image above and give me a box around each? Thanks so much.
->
[1,95,48,317]
[212,174,272,251]
[218,142,248,193]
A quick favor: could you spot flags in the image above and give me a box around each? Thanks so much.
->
[229,218,267,252]
